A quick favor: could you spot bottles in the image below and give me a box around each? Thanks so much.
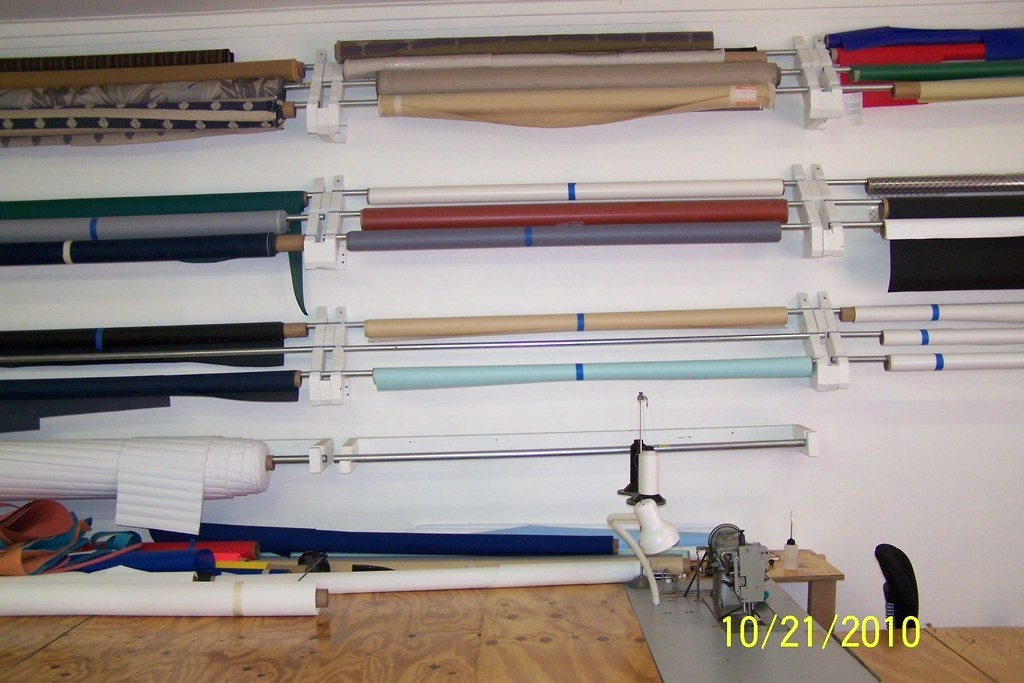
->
[784,537,799,570]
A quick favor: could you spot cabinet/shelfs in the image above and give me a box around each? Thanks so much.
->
[0,0,1024,421]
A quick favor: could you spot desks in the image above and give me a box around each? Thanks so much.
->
[0,527,1023,683]
